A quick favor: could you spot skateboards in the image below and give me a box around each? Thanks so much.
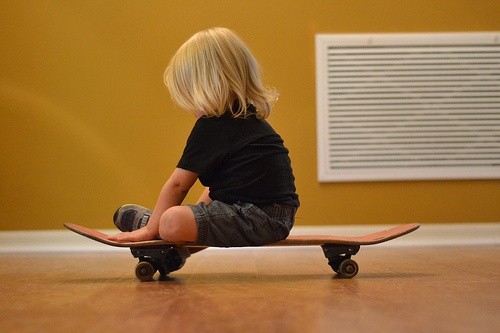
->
[60,220,419,280]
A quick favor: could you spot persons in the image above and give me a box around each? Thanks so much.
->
[107,25,301,272]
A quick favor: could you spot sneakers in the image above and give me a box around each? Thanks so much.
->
[131,246,185,272]
[113,203,152,232]
